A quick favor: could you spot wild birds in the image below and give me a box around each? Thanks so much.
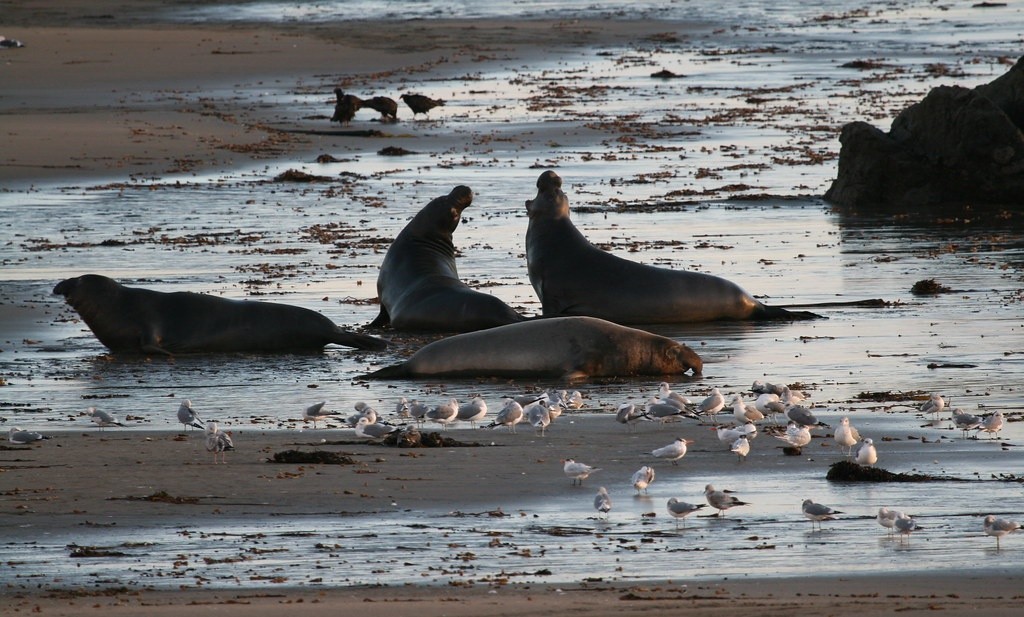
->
[329,88,364,126]
[177,399,206,431]
[9,427,53,444]
[361,96,398,122]
[87,407,128,431]
[563,458,602,487]
[652,438,695,465]
[346,389,584,448]
[593,486,612,518]
[616,382,1022,550]
[398,94,447,121]
[666,498,709,527]
[631,465,655,495]
[302,401,341,428]
[205,422,234,464]
